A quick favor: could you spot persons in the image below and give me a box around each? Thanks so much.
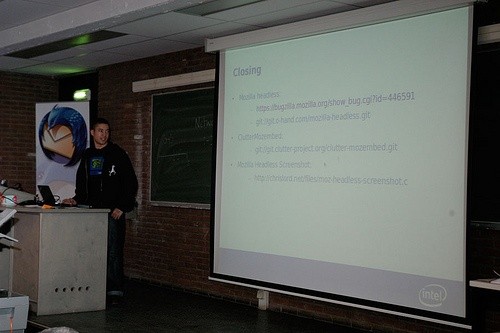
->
[61,117,139,305]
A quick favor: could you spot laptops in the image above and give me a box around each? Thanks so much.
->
[37,185,77,207]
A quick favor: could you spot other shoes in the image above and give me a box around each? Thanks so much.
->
[110,294,124,307]
